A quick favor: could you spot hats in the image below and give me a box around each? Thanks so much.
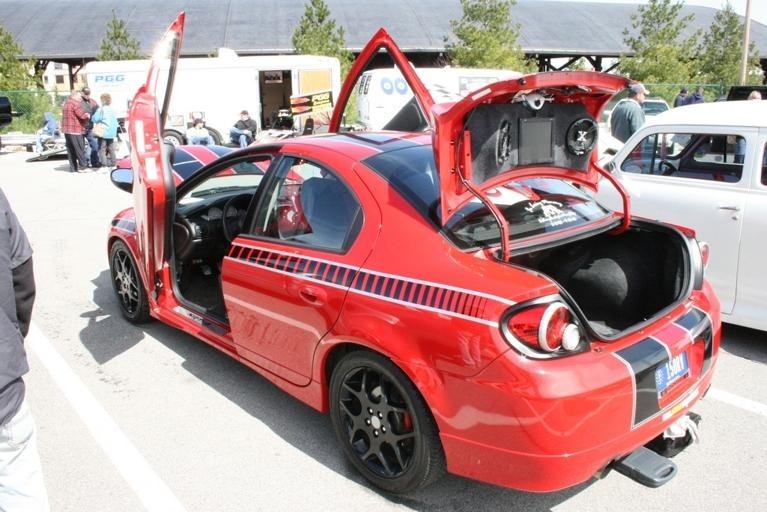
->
[82,87,90,95]
[630,84,650,94]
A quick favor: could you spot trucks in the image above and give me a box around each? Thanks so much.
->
[351,61,527,142]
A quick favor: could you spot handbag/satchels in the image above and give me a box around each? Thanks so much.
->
[92,123,103,138]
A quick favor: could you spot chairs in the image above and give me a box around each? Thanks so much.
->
[278,176,358,251]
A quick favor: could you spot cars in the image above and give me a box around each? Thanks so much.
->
[638,97,671,126]
[99,8,725,507]
[588,93,767,341]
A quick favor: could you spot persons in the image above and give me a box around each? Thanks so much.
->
[733,91,762,164]
[184,119,210,146]
[674,88,688,108]
[684,86,704,105]
[0,189,49,512]
[35,87,127,172]
[230,111,257,148]
[610,83,650,144]
